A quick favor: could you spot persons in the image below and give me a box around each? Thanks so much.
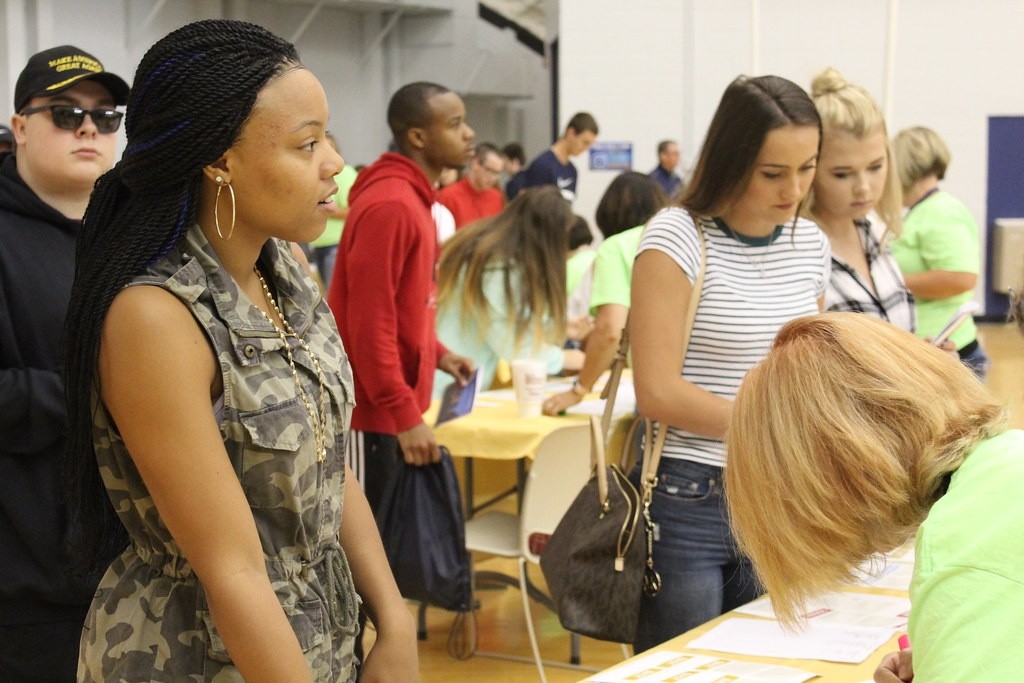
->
[725,311,1024,682]
[0,45,132,683]
[66,20,418,683]
[294,66,987,660]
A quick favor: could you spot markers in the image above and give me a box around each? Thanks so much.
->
[898,634,910,650]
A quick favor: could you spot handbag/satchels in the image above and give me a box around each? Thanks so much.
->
[378,446,475,611]
[540,463,645,644]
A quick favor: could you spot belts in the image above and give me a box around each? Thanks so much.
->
[954,340,978,360]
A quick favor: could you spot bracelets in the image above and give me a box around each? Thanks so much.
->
[573,381,587,396]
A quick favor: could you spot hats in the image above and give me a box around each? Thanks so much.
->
[14,46,131,112]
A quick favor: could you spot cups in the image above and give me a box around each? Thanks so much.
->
[511,359,548,418]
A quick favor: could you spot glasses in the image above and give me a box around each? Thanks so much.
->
[19,105,123,133]
[482,163,503,179]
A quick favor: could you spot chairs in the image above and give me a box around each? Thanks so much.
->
[464,421,605,682]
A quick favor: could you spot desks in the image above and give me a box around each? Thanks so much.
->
[571,535,916,683]
[415,367,637,667]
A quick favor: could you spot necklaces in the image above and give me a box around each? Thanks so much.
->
[729,226,773,275]
[248,265,327,463]
